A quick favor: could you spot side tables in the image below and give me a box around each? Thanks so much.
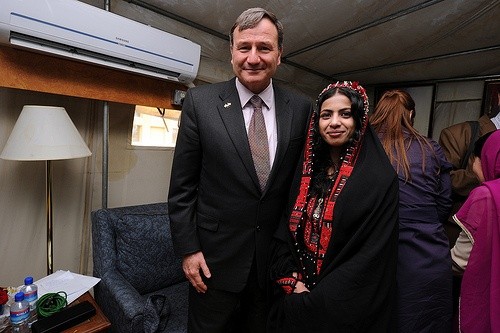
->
[0,289,111,333]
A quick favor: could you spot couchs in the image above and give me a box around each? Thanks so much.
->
[90,202,189,333]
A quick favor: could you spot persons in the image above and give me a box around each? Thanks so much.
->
[167,8,318,333]
[369,86,500,333]
[262,79,401,333]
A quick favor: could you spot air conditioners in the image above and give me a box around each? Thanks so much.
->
[0,0,201,84]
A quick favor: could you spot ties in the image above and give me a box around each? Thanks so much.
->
[247,93,271,193]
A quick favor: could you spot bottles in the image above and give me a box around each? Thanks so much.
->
[20,277,39,329]
[10,292,30,333]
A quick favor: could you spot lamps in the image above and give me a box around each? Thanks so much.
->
[0,105,91,278]
